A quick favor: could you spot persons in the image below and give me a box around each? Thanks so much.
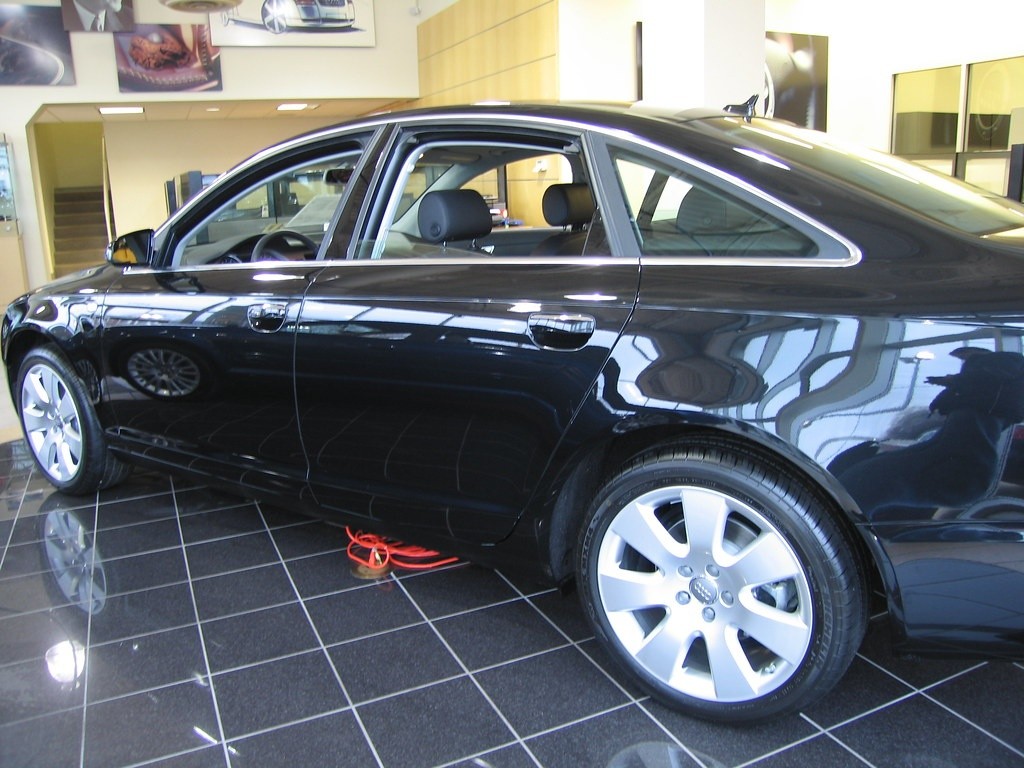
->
[62,0,133,32]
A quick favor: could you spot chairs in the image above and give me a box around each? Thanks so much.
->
[417,188,493,255]
[527,182,597,255]
[676,187,757,231]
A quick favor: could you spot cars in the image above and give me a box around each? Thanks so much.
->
[0,101,1024,728]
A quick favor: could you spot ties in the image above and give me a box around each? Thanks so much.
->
[88,13,103,31]
